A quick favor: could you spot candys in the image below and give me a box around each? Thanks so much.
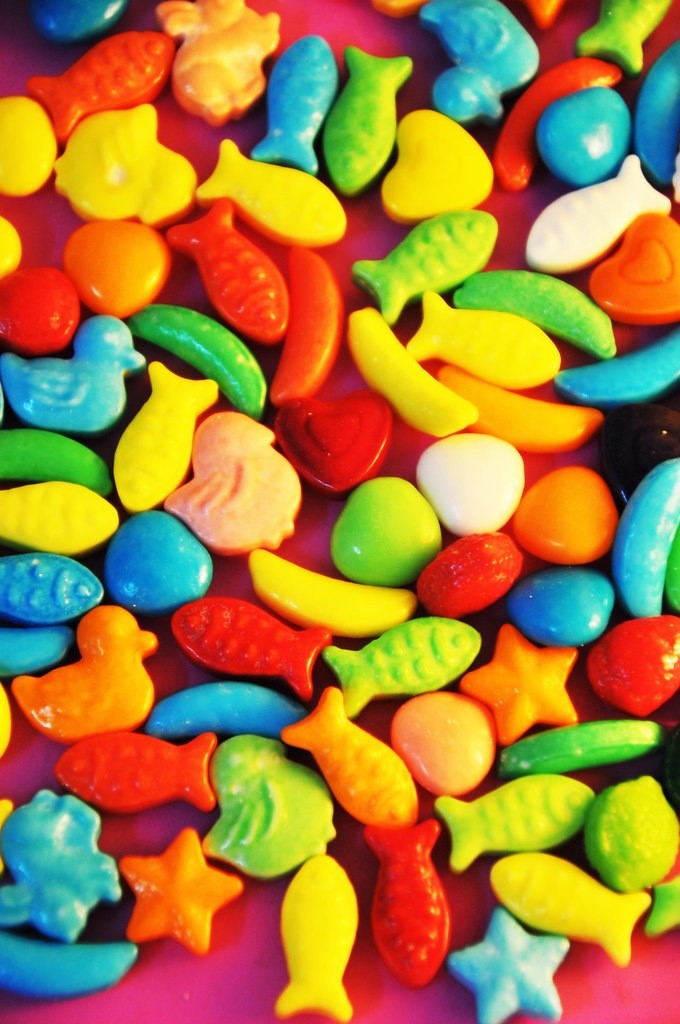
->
[1,1,680,1024]
